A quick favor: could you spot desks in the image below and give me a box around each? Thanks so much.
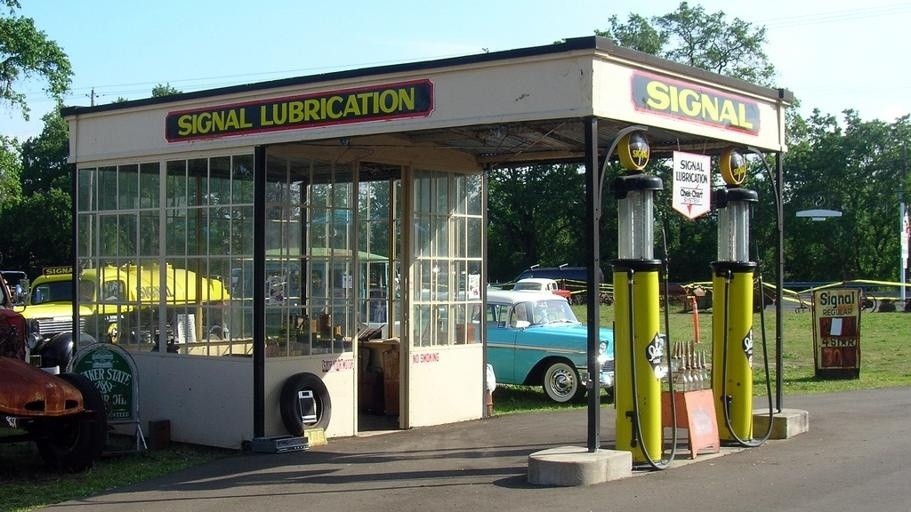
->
[297,337,400,414]
